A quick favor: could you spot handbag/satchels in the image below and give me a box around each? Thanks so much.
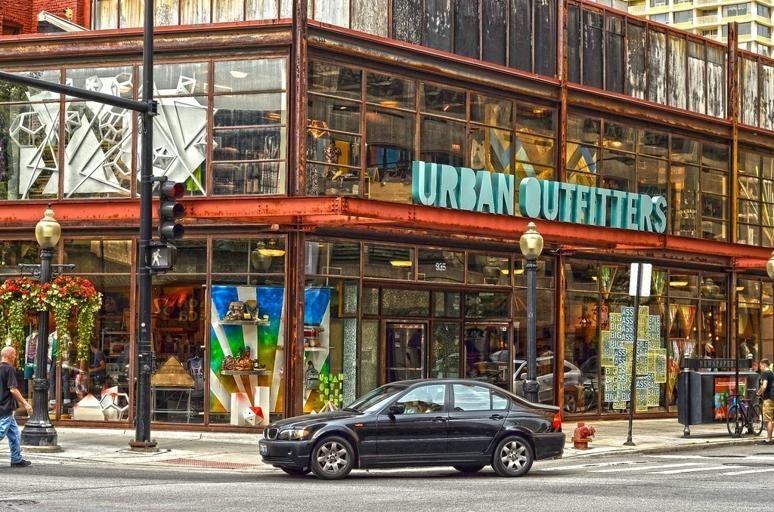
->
[227,299,256,319]
[221,355,254,371]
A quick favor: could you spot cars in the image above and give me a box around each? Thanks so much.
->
[256,377,567,477]
[435,350,666,412]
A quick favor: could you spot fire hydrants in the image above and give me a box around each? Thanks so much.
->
[574,422,594,449]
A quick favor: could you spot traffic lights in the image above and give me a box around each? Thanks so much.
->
[157,176,185,243]
[144,242,177,272]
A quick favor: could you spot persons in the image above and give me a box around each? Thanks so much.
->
[704,336,719,372]
[23,328,40,383]
[413,391,433,414]
[0,346,33,467]
[402,394,418,414]
[756,358,773,443]
[536,337,548,355]
[74,355,90,400]
[48,326,72,407]
[88,342,105,401]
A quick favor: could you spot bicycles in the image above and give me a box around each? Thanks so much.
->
[726,387,763,437]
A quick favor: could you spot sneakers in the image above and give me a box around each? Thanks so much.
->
[11,460,31,466]
[757,440,773,445]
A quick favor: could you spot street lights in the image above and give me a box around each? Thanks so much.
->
[701,276,719,360]
[19,199,66,446]
[567,154,636,179]
[766,251,773,369]
[517,216,544,404]
[252,240,274,288]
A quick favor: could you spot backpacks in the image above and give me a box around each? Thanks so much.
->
[306,368,320,390]
